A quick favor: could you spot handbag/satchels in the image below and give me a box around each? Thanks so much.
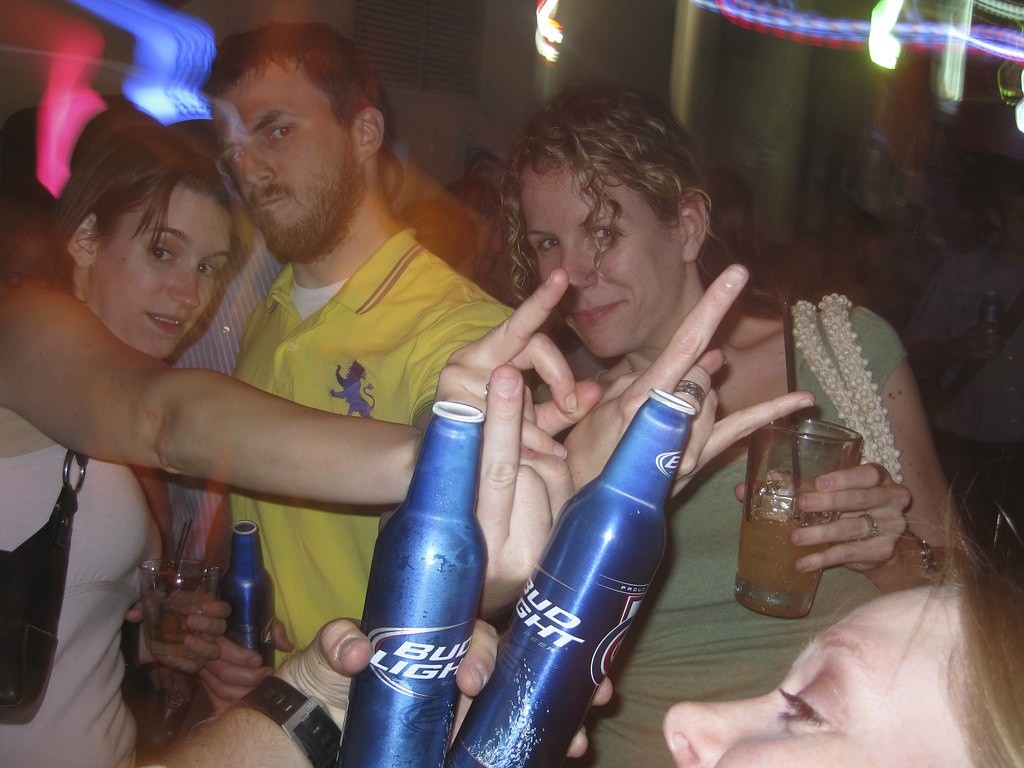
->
[0,449,88,726]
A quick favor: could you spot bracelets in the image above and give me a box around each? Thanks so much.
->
[241,674,342,768]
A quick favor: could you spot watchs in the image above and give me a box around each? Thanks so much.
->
[915,535,936,586]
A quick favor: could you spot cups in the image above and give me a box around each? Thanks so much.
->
[141,560,222,657]
[737,414,863,617]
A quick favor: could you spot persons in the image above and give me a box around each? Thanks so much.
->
[0,16,1024,768]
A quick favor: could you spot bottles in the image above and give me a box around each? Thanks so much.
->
[340,401,488,768]
[147,672,196,747]
[444,389,695,768]
[979,288,1003,356]
[219,520,275,669]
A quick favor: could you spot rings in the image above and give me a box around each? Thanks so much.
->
[674,379,705,410]
[484,383,491,401]
[860,514,878,541]
